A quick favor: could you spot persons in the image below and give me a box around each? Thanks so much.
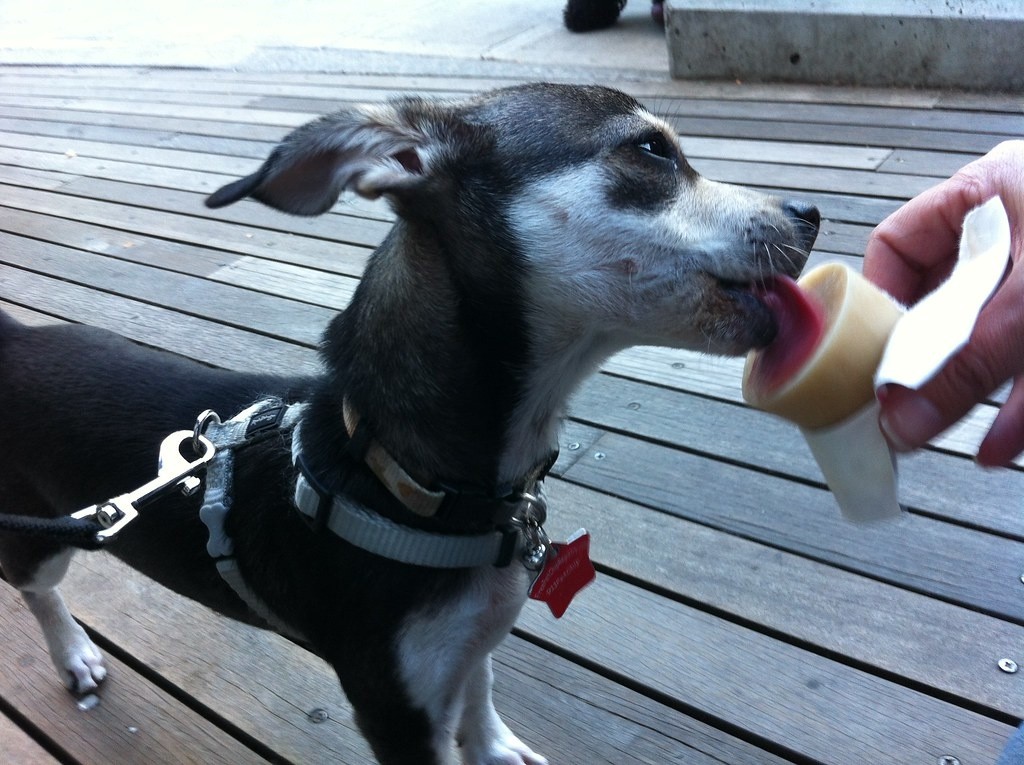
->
[864,140,1024,467]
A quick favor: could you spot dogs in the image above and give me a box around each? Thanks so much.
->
[0,80,822,765]
